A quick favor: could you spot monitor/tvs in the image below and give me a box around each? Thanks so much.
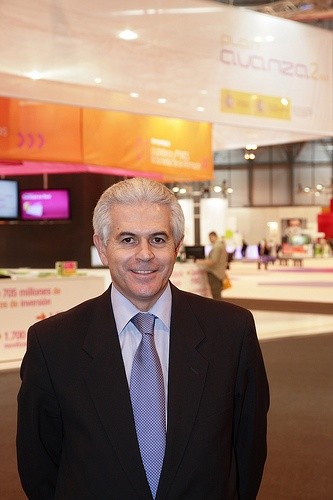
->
[185,247,204,259]
[0,179,19,221]
[19,188,71,221]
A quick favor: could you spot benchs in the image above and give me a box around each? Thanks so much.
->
[278,257,303,267]
[257,258,275,270]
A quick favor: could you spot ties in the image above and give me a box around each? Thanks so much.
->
[129,313,166,500]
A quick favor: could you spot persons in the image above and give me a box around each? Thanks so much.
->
[237,237,279,259]
[15,176,271,500]
[196,231,228,299]
